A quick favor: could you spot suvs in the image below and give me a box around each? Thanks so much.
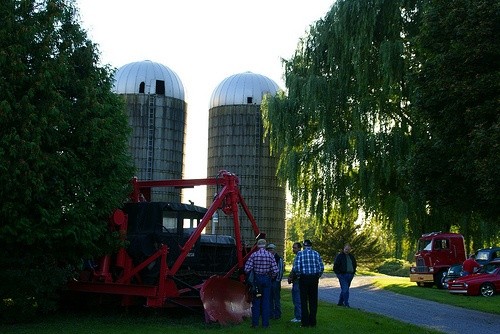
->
[442,247,500,288]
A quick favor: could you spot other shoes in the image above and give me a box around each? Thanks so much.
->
[291,318,301,322]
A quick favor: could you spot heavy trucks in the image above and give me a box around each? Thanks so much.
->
[410,232,467,288]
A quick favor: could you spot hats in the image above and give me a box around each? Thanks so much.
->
[303,240,312,246]
[267,244,276,250]
[257,239,266,247]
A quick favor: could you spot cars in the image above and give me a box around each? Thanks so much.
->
[446,260,500,298]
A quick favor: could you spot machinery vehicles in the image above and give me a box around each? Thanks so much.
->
[69,170,266,326]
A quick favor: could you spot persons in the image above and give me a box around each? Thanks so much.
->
[333,246,357,307]
[245,239,324,329]
[462,255,481,277]
